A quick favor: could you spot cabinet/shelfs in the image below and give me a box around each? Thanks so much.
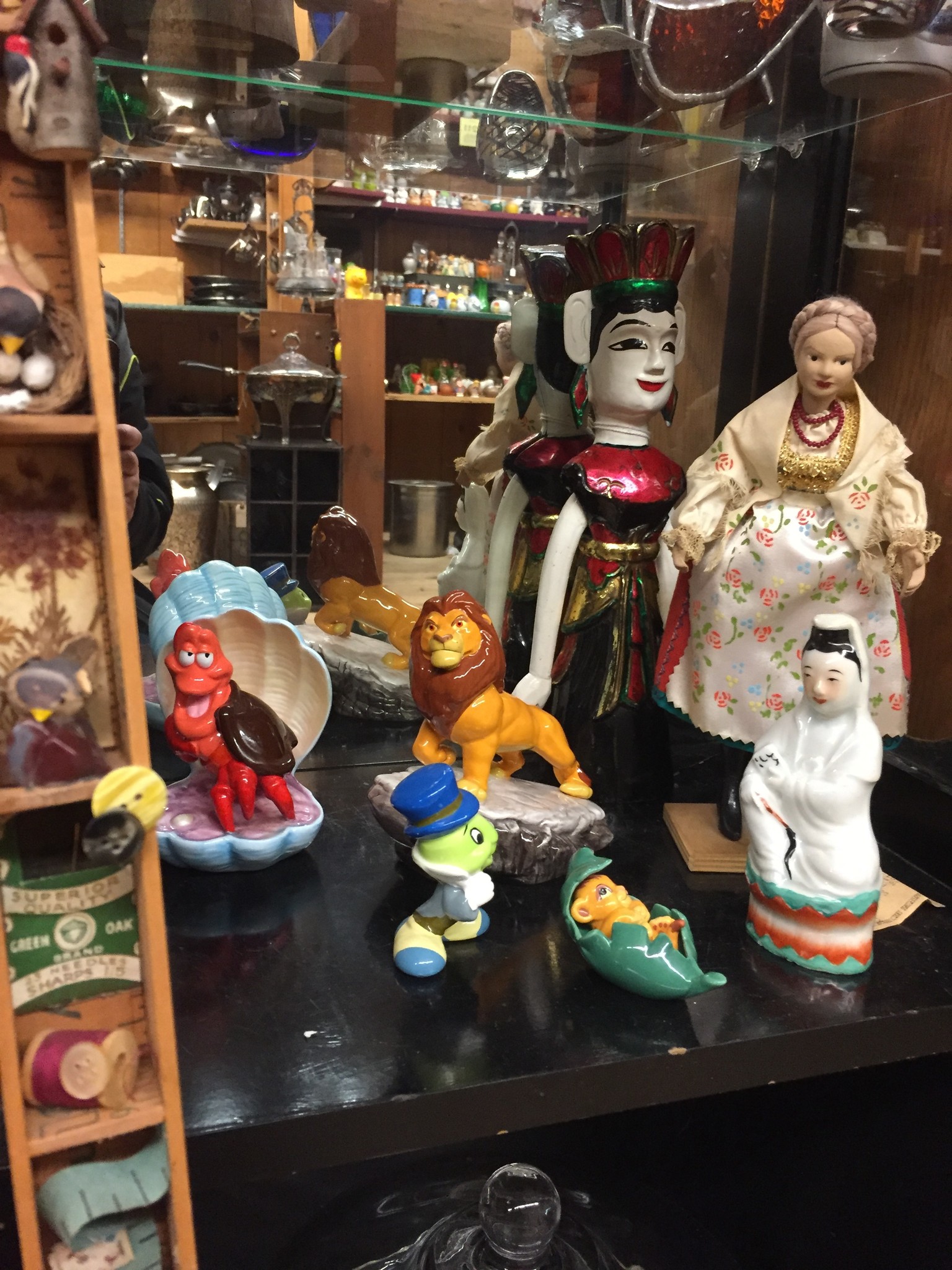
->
[384,303,512,405]
[318,298,384,586]
[265,173,315,310]
[245,438,348,603]
[237,309,331,439]
[0,161,202,1270]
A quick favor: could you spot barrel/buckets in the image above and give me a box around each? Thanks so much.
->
[388,480,457,558]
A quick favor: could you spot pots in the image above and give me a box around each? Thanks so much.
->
[178,332,347,404]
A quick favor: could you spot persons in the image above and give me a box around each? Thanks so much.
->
[486,243,594,683]
[437,323,540,609]
[101,293,173,568]
[510,221,696,718]
[736,612,884,899]
[650,296,942,745]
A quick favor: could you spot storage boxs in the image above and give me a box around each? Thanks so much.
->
[407,274,474,295]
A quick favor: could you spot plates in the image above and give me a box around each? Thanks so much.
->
[188,274,261,310]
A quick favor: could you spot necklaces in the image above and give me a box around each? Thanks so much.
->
[592,421,649,440]
[540,414,576,427]
[776,396,855,491]
